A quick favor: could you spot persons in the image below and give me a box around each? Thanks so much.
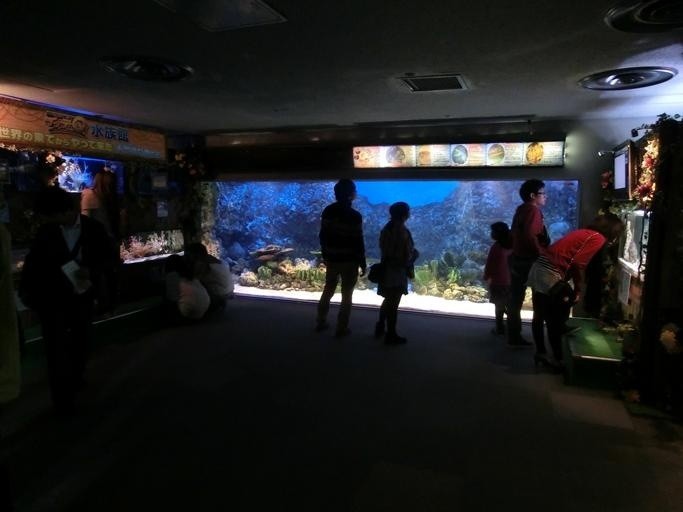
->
[16,182,115,424]
[525,213,626,376]
[371,202,416,345]
[312,177,367,338]
[502,178,552,349]
[482,221,523,336]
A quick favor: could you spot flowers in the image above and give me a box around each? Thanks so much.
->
[628,138,661,210]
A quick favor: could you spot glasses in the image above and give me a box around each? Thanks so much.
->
[537,191,545,195]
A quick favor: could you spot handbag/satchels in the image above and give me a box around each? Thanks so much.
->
[368,262,382,283]
[548,279,576,309]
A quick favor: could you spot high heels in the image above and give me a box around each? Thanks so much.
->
[533,354,557,370]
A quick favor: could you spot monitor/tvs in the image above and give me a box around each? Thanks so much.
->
[610,140,638,201]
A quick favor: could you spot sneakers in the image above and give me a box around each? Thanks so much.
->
[384,334,408,344]
[506,337,534,349]
[315,322,332,332]
[335,328,353,336]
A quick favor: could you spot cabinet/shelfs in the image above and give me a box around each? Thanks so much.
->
[601,117,682,416]
